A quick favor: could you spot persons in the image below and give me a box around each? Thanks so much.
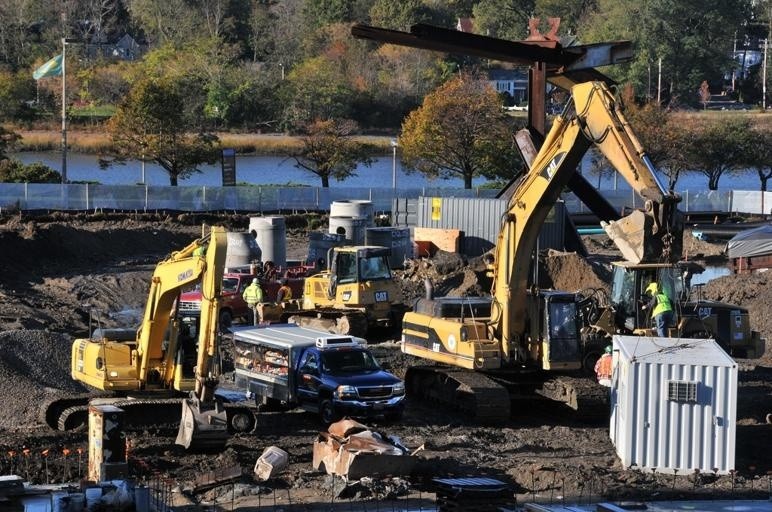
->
[276,277,293,303]
[243,277,264,326]
[592,345,613,401]
[641,282,674,338]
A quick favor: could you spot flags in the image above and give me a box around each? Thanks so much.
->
[33,54,65,80]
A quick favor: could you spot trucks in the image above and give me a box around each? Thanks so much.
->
[180,263,316,329]
[232,322,406,427]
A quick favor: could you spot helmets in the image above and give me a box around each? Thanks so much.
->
[252,277,260,284]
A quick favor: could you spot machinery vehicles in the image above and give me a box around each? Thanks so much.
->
[255,245,402,336]
[39,226,258,436]
